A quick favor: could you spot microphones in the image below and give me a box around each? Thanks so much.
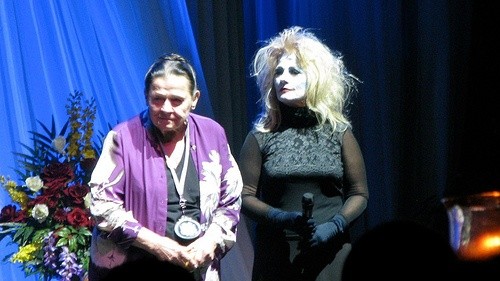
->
[301,192,315,251]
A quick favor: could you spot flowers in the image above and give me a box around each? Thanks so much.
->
[0,89,121,281]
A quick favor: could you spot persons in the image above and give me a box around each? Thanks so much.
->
[340,220,461,281]
[97,255,195,281]
[237,26,370,281]
[88,53,243,281]
[436,174,500,260]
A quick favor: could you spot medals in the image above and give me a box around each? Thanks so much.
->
[174,215,202,240]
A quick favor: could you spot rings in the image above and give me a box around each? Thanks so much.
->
[184,260,190,268]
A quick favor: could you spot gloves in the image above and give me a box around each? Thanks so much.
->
[300,214,347,249]
[268,208,317,239]
